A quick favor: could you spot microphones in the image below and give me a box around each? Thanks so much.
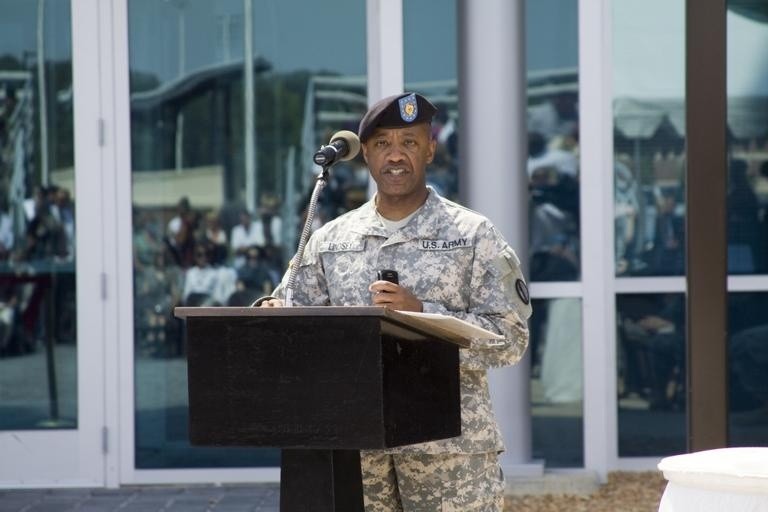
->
[315,130,362,168]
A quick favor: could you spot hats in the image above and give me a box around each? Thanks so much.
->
[358,92,438,143]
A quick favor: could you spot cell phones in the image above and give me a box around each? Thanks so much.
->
[377,270,398,293]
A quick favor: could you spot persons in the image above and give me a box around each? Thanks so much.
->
[133,176,380,362]
[0,175,76,353]
[429,103,456,203]
[529,157,767,412]
[250,92,533,512]
